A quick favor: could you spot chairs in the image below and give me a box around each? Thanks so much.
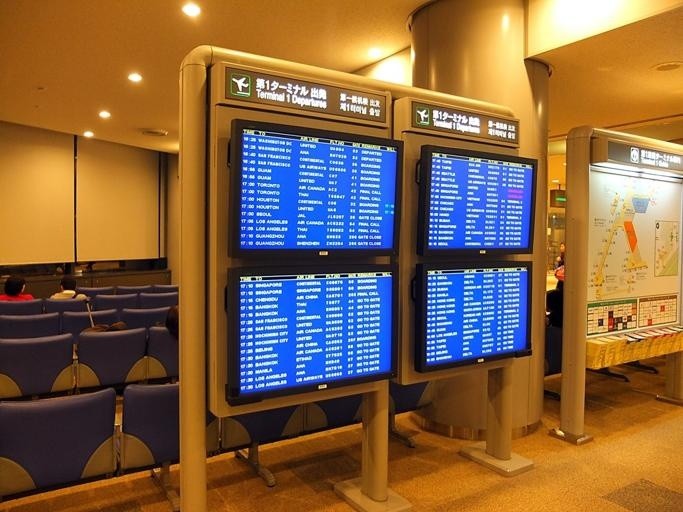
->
[390,382,434,446]
[1,284,177,508]
[206,394,364,485]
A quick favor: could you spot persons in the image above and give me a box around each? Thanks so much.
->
[0,275,34,302]
[50,276,87,301]
[554,241,566,291]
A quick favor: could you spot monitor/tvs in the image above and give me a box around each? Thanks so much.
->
[226,263,400,407]
[228,118,404,257]
[415,261,533,373]
[416,145,538,256]
